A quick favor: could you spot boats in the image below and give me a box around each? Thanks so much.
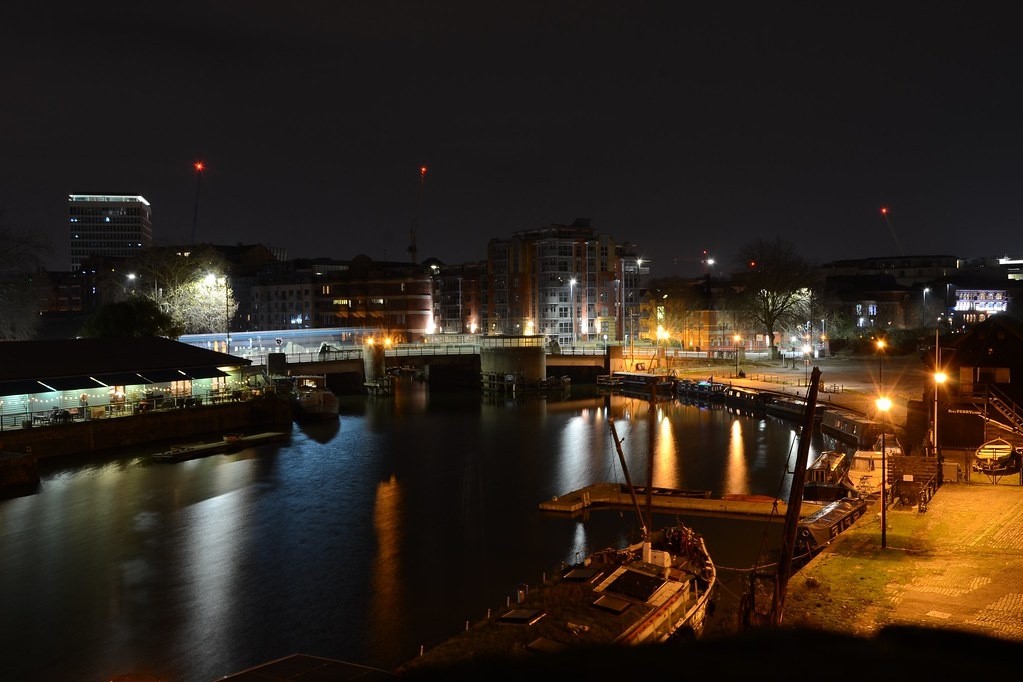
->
[376,413,716,682]
[291,374,339,415]
[975,437,1014,471]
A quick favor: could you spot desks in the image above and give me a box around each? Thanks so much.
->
[34,415,46,425]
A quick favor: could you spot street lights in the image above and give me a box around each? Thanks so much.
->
[622,257,642,350]
[876,341,884,397]
[734,335,740,377]
[821,319,825,346]
[877,398,894,549]
[206,273,230,354]
[791,336,797,369]
[806,321,811,346]
[570,278,576,345]
[933,372,947,452]
[804,347,811,387]
